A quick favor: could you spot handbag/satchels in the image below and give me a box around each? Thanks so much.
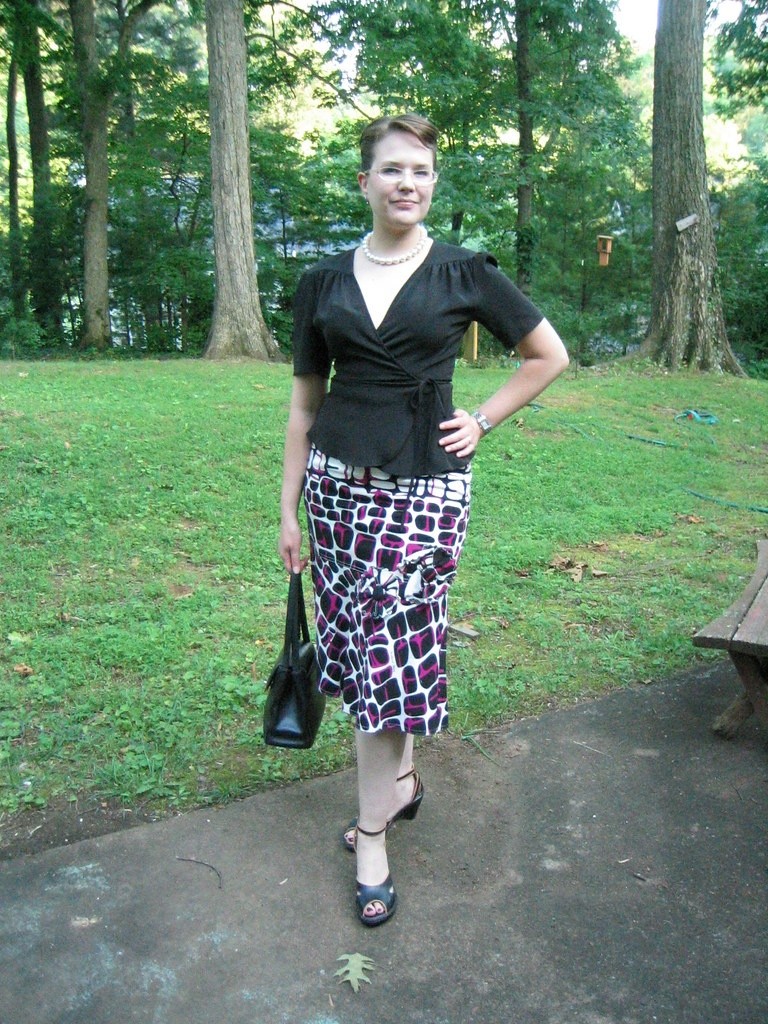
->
[262,570,326,749]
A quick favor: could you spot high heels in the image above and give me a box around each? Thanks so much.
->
[353,819,397,926]
[343,763,425,851]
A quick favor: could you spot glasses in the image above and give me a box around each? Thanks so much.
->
[364,166,438,185]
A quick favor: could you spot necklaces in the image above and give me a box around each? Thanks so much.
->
[362,226,427,265]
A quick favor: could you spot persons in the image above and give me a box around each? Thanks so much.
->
[278,114,570,924]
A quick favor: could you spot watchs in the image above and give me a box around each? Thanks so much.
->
[471,412,491,435]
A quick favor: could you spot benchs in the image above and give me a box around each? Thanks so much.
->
[692,539,768,741]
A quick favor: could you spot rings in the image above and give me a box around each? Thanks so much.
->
[466,437,471,444]
